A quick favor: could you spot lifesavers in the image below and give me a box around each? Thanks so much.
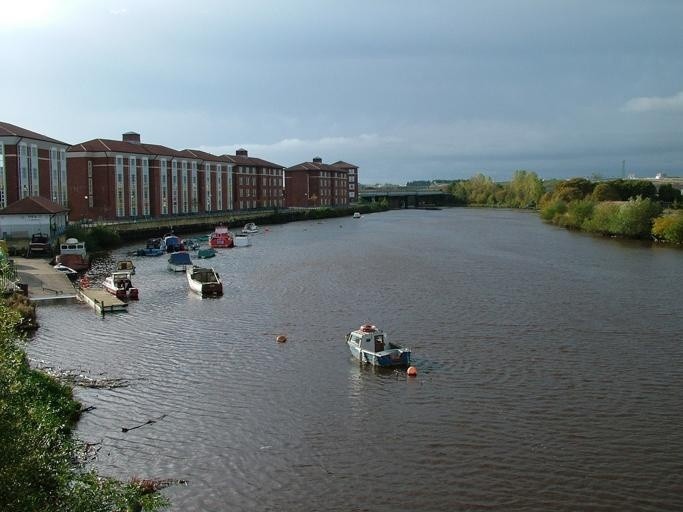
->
[360,324,376,332]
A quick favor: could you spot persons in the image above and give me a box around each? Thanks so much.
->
[126,279,132,290]
[118,280,125,288]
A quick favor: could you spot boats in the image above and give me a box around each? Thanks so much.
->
[185,264,223,297]
[53,264,77,277]
[113,257,136,275]
[353,212,361,218]
[56,237,93,271]
[102,271,139,301]
[136,221,259,271]
[344,323,411,368]
